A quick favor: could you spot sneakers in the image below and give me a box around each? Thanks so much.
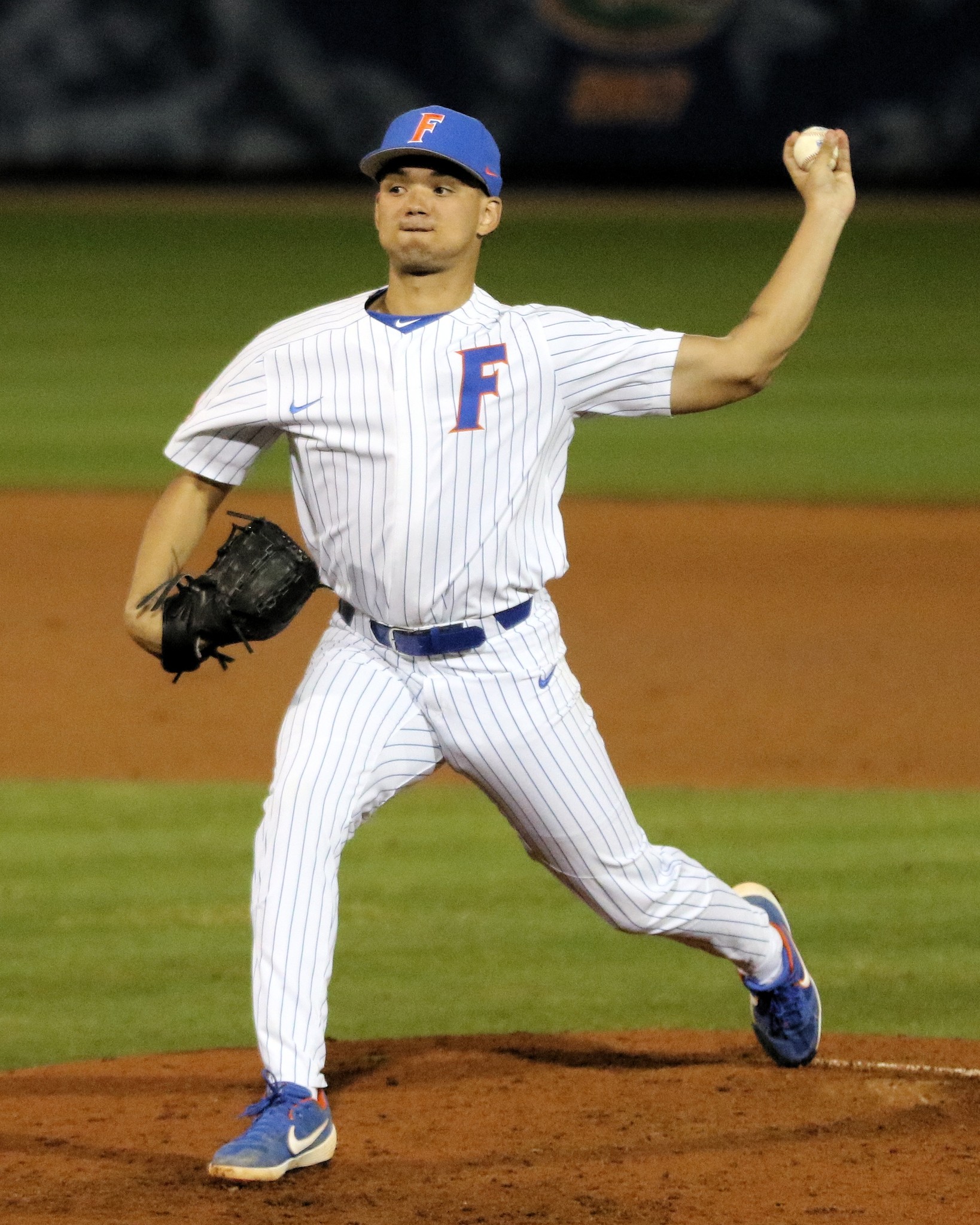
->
[209,1070,337,1181]
[731,881,822,1067]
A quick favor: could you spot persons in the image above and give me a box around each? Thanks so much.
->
[123,106,857,1183]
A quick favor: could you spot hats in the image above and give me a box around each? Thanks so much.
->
[359,106,503,197]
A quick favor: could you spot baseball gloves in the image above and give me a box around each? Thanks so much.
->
[135,509,320,684]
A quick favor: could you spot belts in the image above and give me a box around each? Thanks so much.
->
[337,598,532,656]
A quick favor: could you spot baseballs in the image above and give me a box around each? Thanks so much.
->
[793,125,839,172]
